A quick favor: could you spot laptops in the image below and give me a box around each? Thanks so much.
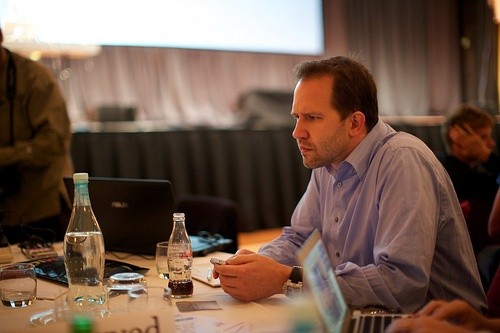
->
[293,228,414,333]
[62,176,233,256]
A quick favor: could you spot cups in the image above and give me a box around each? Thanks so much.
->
[0,263,38,308]
[128,286,179,316]
[156,242,168,280]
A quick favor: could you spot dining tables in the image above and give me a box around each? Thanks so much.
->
[0,254,324,333]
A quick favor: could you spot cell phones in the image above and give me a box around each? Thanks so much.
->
[210,258,228,265]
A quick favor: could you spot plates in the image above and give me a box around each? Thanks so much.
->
[29,308,111,324]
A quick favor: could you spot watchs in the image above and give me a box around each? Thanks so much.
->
[282,265,303,299]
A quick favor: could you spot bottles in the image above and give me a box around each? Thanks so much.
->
[167,212,193,298]
[63,173,106,308]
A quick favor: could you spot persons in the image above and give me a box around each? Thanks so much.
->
[0,25,75,249]
[212,52,500,333]
